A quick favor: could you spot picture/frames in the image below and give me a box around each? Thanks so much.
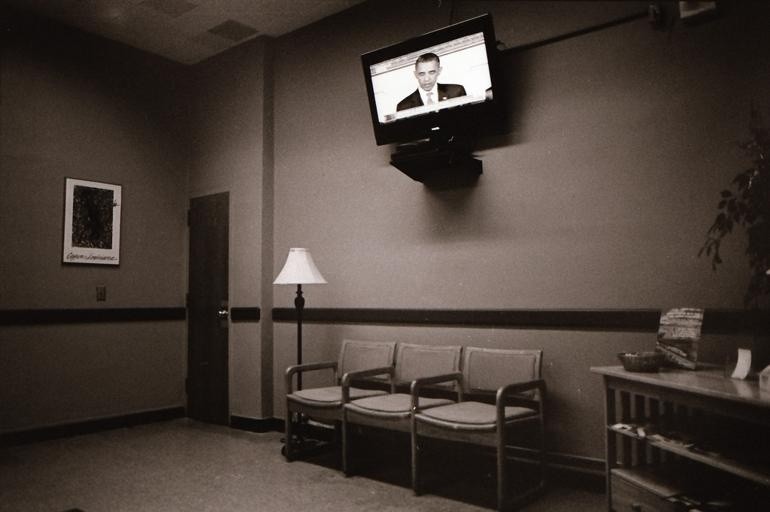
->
[62,176,123,268]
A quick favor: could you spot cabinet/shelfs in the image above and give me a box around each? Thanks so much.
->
[590,361,770,512]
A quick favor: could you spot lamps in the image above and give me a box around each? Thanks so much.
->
[272,248,329,441]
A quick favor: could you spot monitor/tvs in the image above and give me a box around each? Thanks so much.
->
[359,11,503,146]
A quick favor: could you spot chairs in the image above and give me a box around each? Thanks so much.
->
[283,340,550,512]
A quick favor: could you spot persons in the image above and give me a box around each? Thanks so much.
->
[396,52,466,113]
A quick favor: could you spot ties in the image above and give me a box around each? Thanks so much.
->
[424,91,434,106]
